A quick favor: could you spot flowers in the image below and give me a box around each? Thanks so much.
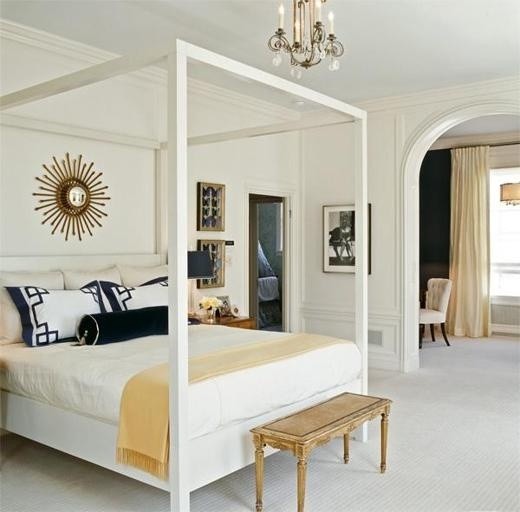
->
[195,297,227,315]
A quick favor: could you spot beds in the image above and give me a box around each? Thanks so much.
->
[1,37,369,512]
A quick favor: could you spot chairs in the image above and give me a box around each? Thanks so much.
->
[418,277,455,349]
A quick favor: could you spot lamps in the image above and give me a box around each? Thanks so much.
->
[499,182,520,205]
[268,0,345,81]
[188,250,214,320]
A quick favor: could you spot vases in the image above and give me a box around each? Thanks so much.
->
[205,310,216,324]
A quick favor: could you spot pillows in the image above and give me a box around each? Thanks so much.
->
[1,269,65,343]
[97,276,196,325]
[61,267,121,292]
[5,280,106,351]
[74,304,167,346]
[117,262,170,293]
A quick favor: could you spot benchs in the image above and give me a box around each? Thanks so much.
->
[250,391,395,512]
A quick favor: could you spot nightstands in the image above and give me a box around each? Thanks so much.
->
[209,315,256,329]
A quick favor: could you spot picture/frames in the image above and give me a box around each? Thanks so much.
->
[198,181,225,231]
[322,203,370,273]
[196,239,225,288]
